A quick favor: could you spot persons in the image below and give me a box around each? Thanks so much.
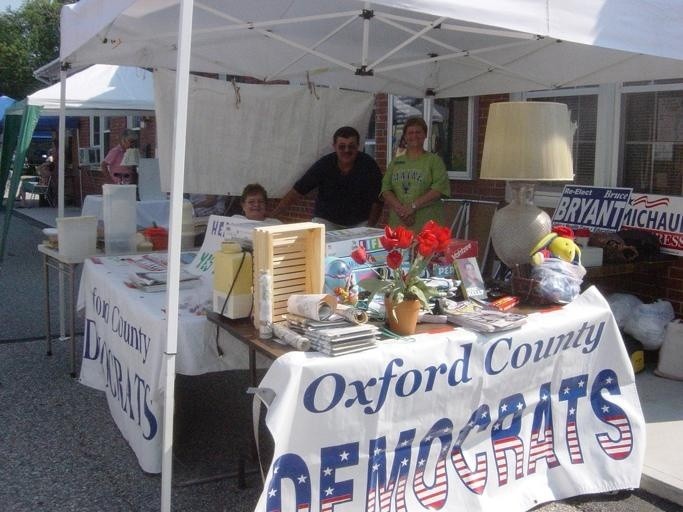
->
[98,126,142,185]
[265,126,382,228]
[226,182,284,225]
[15,160,55,207]
[376,117,452,233]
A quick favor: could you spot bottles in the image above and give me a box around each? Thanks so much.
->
[574,228,589,248]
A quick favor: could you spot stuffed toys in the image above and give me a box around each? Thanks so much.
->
[533,221,584,266]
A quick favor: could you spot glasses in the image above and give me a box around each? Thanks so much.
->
[337,143,356,151]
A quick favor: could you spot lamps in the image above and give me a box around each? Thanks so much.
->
[478,102,575,269]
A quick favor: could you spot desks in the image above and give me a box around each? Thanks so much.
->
[16,175,38,199]
[36,243,102,376]
[75,250,277,476]
[207,283,646,512]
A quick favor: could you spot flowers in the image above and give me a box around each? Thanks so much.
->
[351,220,452,323]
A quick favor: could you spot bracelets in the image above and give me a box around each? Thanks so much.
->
[411,201,417,212]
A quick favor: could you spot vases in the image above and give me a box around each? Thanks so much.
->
[385,293,421,334]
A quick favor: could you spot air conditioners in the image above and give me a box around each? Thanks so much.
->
[78,147,100,167]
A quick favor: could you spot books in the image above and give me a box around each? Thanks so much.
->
[286,309,380,354]
[441,253,530,333]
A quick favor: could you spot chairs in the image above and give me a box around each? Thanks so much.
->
[29,176,56,208]
